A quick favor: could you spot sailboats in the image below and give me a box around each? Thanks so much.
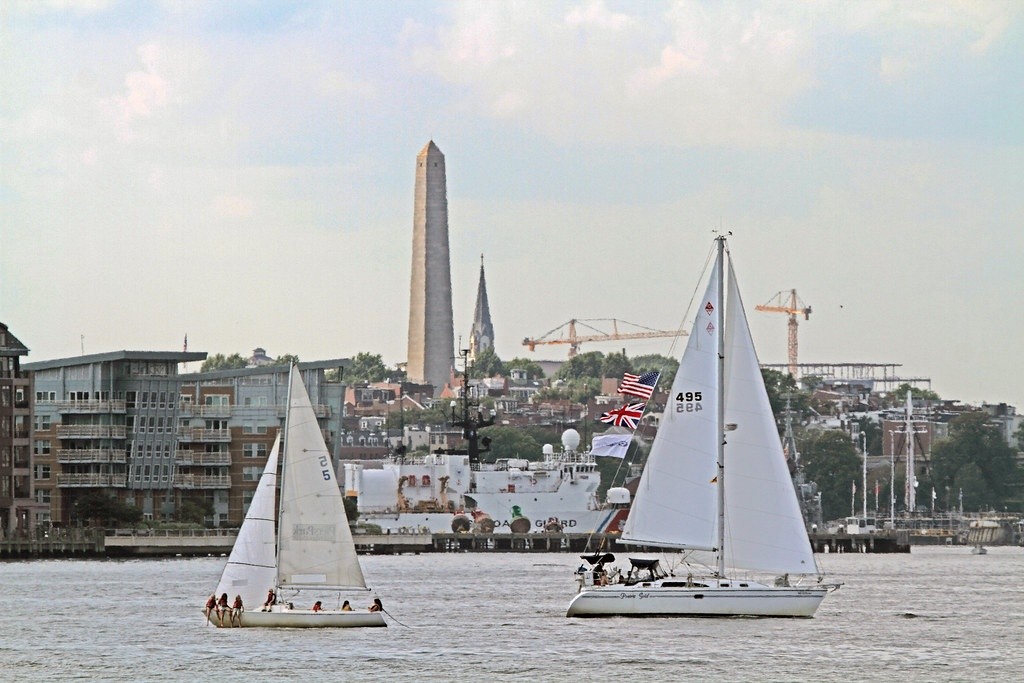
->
[200,354,388,628]
[566,229,844,618]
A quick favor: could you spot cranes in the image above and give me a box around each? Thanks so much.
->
[753,289,812,375]
[520,317,689,360]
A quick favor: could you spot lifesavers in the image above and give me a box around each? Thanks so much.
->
[422,475,430,485]
[408,474,416,486]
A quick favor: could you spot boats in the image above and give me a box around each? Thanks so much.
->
[972,545,987,556]
[344,336,631,534]
[831,388,1023,546]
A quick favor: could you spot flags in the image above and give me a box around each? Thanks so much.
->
[617,371,660,398]
[590,434,632,459]
[598,402,647,430]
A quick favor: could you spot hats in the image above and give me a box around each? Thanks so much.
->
[269,589,273,592]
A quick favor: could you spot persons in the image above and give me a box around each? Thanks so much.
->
[313,601,322,613]
[206,593,244,628]
[600,565,623,586]
[342,600,355,611]
[368,599,382,612]
[262,589,276,612]
[813,524,817,534]
